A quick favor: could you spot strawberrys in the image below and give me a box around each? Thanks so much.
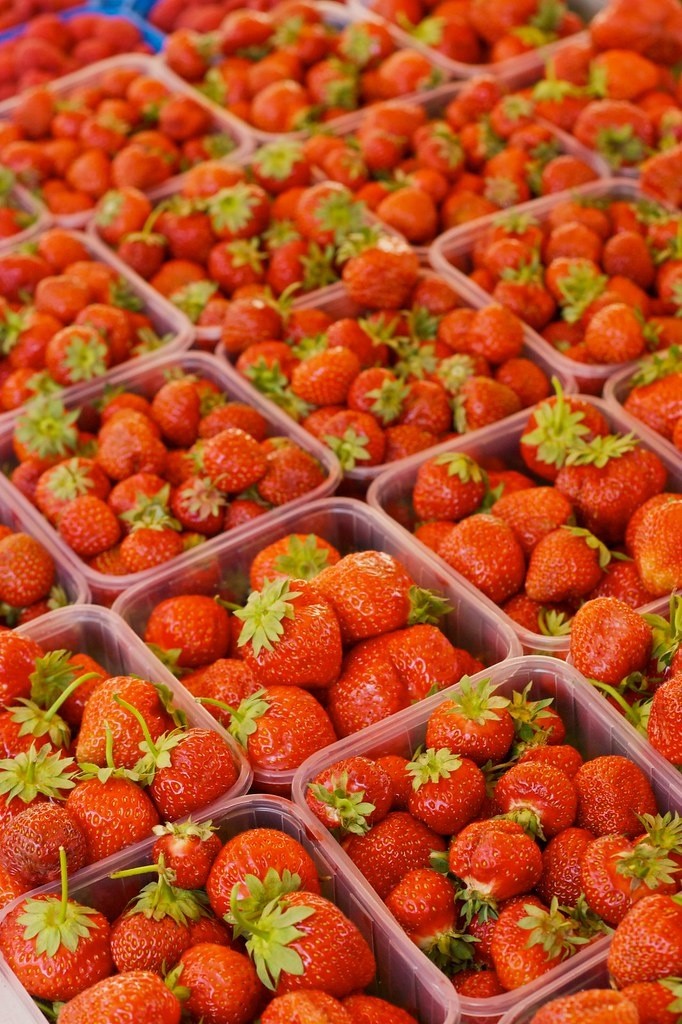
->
[0,0,682,1024]
